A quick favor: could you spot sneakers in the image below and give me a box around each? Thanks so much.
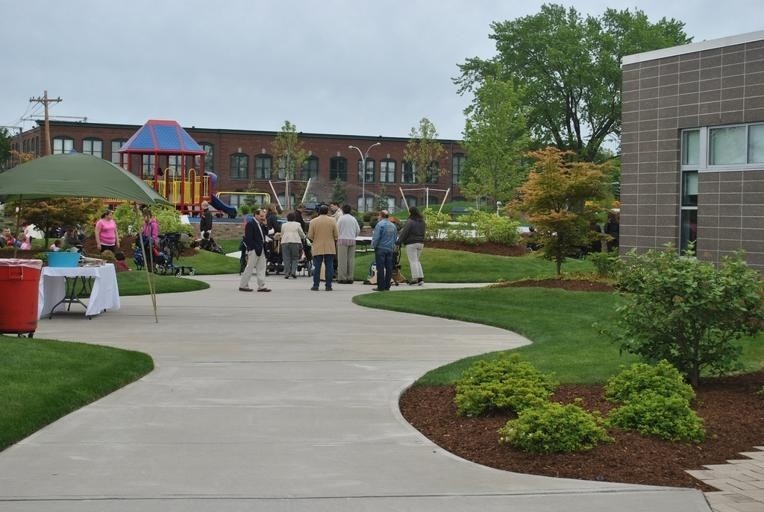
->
[418,276,425,286]
[409,278,419,286]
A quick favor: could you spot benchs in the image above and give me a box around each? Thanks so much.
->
[155,265,195,276]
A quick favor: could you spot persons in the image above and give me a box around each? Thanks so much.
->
[142,210,158,248]
[50,240,62,251]
[200,200,212,241]
[395,207,426,286]
[152,167,164,180]
[17,233,33,251]
[371,210,397,291]
[95,210,120,255]
[307,205,338,291]
[263,201,341,224]
[239,209,272,292]
[336,205,361,284]
[199,232,212,251]
[280,213,306,280]
[0,227,16,248]
[114,251,132,272]
[152,246,168,260]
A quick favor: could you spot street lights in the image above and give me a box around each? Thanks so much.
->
[347,141,382,213]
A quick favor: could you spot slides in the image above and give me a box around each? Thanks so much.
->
[208,193,237,218]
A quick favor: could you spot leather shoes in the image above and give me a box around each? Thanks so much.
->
[325,287,333,291]
[257,288,271,292]
[239,288,253,291]
[311,287,318,291]
[372,287,385,291]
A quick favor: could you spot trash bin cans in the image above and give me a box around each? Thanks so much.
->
[0,258,44,339]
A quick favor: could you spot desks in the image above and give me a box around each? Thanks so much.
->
[41,262,118,317]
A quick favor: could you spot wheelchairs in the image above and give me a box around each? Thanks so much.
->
[363,240,409,286]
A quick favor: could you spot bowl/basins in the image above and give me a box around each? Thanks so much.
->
[45,251,81,268]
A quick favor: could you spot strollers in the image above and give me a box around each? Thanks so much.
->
[288,238,313,277]
[242,236,284,275]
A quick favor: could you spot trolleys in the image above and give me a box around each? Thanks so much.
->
[160,231,199,276]
